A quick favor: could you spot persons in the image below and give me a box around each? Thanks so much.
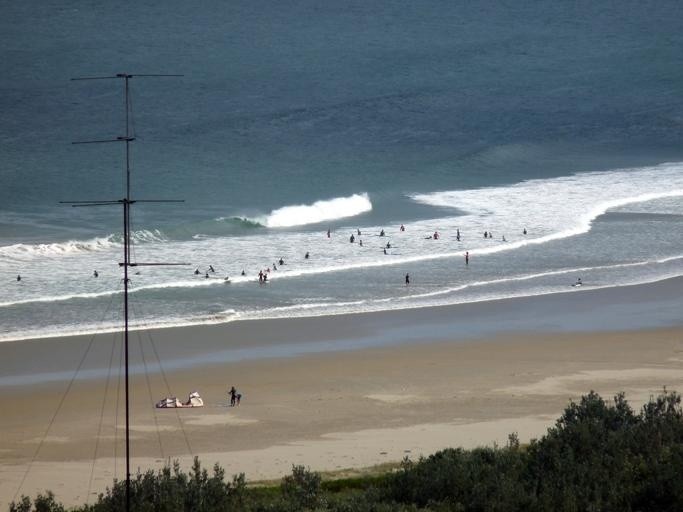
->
[489,232,492,238]
[345,223,407,257]
[405,273,410,284]
[523,228,527,234]
[192,249,311,284]
[456,229,460,241]
[228,386,236,407]
[326,229,332,238]
[16,275,21,281]
[434,232,439,239]
[236,393,241,406]
[93,270,99,278]
[575,278,582,287]
[464,252,469,264]
[484,231,488,237]
[502,235,505,241]
